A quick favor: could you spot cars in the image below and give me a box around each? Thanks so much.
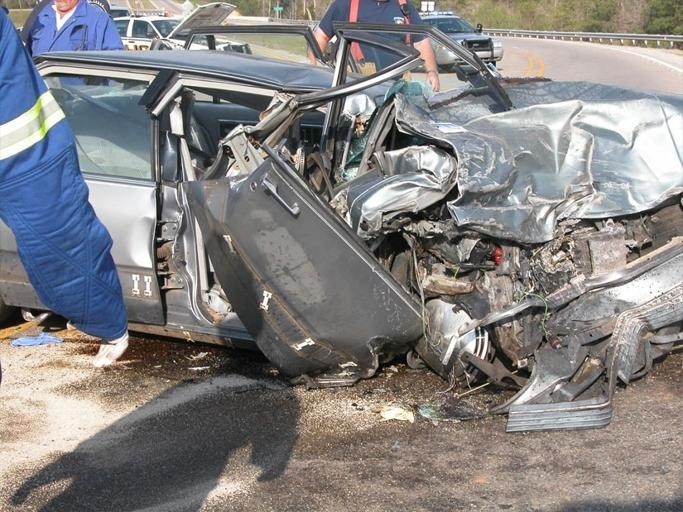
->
[0,50,369,358]
[109,6,130,20]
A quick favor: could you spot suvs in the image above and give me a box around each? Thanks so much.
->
[417,11,506,72]
[113,15,253,56]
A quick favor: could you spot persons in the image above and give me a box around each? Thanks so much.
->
[307,0,440,92]
[0,0,129,368]
[27,0,123,86]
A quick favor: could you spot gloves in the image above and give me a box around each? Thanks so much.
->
[93,329,128,367]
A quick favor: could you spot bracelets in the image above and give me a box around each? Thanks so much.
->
[427,69,438,76]
